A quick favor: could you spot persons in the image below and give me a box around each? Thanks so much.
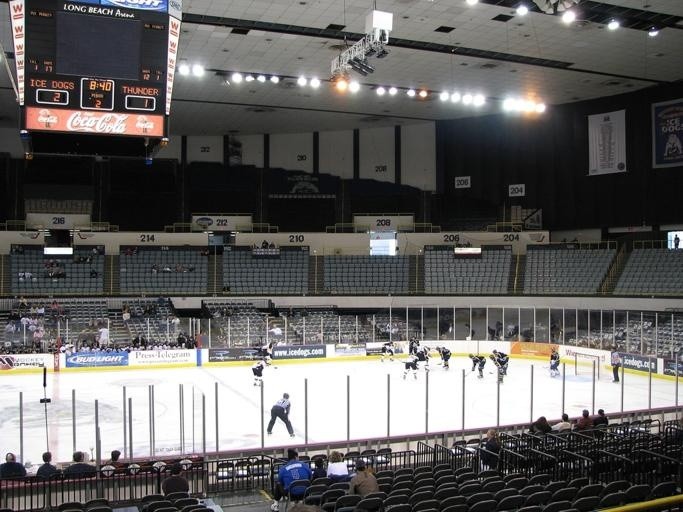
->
[550,414,570,432]
[593,409,608,427]
[266,323,283,345]
[259,343,274,369]
[480,430,500,470]
[528,416,551,434]
[220,305,231,319]
[199,244,210,257]
[380,322,649,384]
[269,241,276,249]
[672,234,679,250]
[212,307,221,320]
[0,295,199,353]
[0,451,28,481]
[222,283,230,292]
[63,450,96,479]
[350,459,380,495]
[250,356,268,384]
[573,409,592,429]
[327,449,348,478]
[19,247,195,282]
[260,239,267,249]
[33,450,60,478]
[102,450,126,478]
[269,448,313,512]
[266,392,294,438]
[161,464,188,496]
[311,454,328,480]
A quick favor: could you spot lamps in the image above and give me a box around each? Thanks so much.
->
[459,0,660,37]
[326,27,396,79]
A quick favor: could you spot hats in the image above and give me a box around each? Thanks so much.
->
[287,448,299,460]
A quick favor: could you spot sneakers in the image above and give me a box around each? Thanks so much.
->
[270,502,279,512]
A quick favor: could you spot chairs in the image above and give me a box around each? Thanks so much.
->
[424,242,683,366]
[272,419,682,512]
[213,460,271,493]
[22,458,213,512]
[0,239,418,353]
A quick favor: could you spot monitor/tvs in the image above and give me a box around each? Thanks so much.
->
[208,230,237,246]
[44,229,74,247]
